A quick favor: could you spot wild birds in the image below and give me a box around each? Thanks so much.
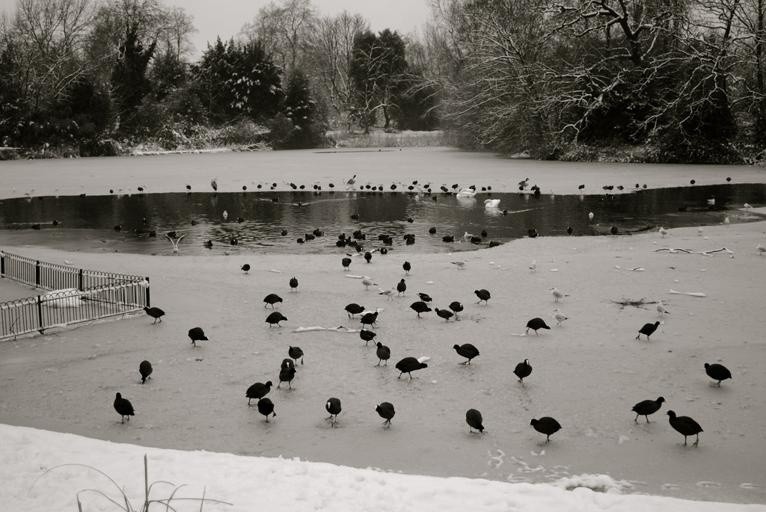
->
[241,263,250,273]
[404,261,411,274]
[143,306,165,325]
[656,298,670,317]
[113,392,134,425]
[665,410,704,448]
[704,362,732,387]
[364,252,372,264]
[342,258,351,270]
[188,327,208,347]
[635,321,660,342]
[245,276,570,443]
[23,174,766,255]
[138,360,153,384]
[631,396,666,425]
